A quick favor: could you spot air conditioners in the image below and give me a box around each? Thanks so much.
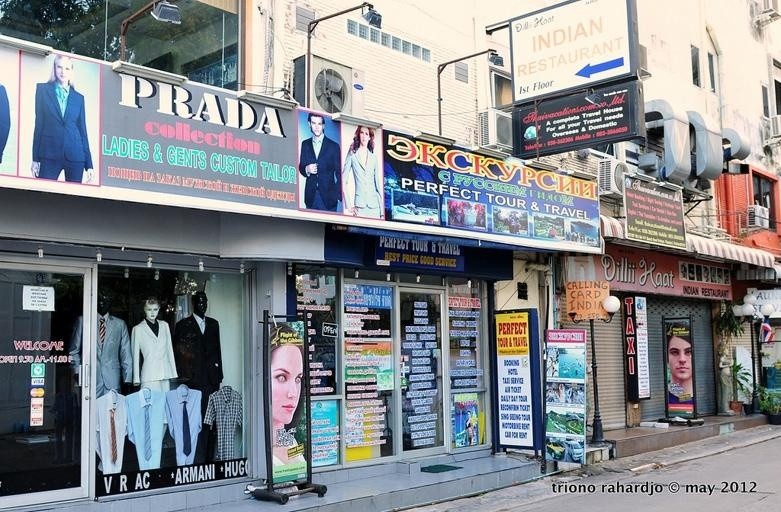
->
[293,52,353,117]
[598,160,638,205]
[746,205,770,229]
[477,109,514,157]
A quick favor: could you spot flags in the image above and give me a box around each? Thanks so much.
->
[761,318,775,347]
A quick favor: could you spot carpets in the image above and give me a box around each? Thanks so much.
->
[421,464,463,473]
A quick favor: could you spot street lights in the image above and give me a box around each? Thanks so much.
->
[569,296,621,445]
[733,293,773,413]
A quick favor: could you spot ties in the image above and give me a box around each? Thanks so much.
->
[144,405,152,460]
[182,401,191,456]
[110,408,117,464]
[99,317,106,344]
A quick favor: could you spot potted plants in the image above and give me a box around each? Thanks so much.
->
[729,356,781,424]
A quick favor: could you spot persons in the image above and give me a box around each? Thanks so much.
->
[342,126,386,221]
[129,296,177,456]
[298,114,342,212]
[717,343,735,413]
[465,411,472,445]
[666,323,694,403]
[174,290,223,457]
[30,54,95,184]
[65,284,132,472]
[267,322,306,468]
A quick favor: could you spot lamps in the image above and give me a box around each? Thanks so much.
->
[121,0,181,62]
[307,3,382,107]
[437,49,504,135]
